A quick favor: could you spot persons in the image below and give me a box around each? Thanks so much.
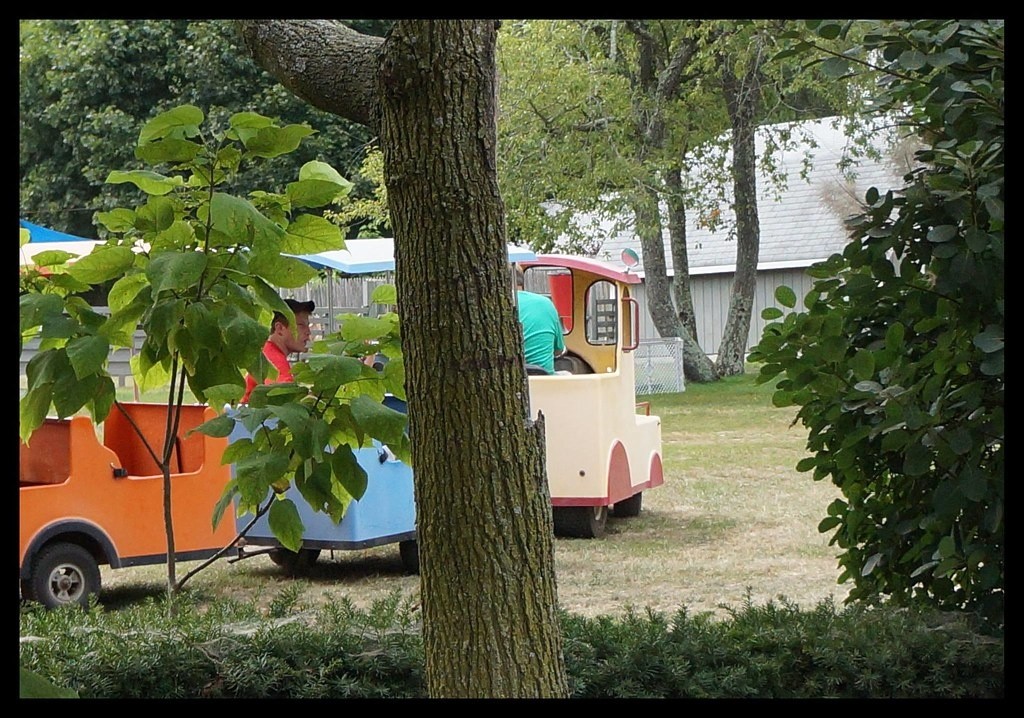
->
[508,264,564,376]
[240,299,374,404]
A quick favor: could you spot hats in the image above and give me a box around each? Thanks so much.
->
[273,299,315,321]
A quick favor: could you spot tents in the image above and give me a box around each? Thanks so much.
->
[19,219,151,278]
